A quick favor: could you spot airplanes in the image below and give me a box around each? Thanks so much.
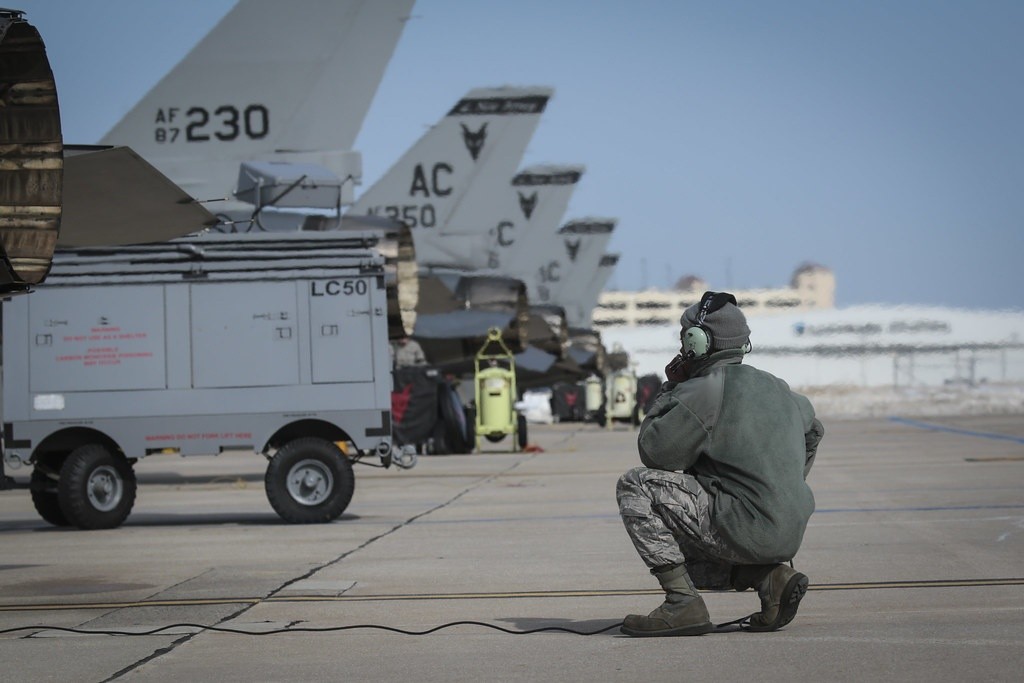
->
[0,0,631,387]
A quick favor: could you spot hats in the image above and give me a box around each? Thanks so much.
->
[680,301,751,350]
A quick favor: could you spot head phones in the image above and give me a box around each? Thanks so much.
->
[683,291,749,358]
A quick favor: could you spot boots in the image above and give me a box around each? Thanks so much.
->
[746,563,809,631]
[620,562,713,637]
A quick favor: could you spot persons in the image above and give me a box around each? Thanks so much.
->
[616,291,825,636]
